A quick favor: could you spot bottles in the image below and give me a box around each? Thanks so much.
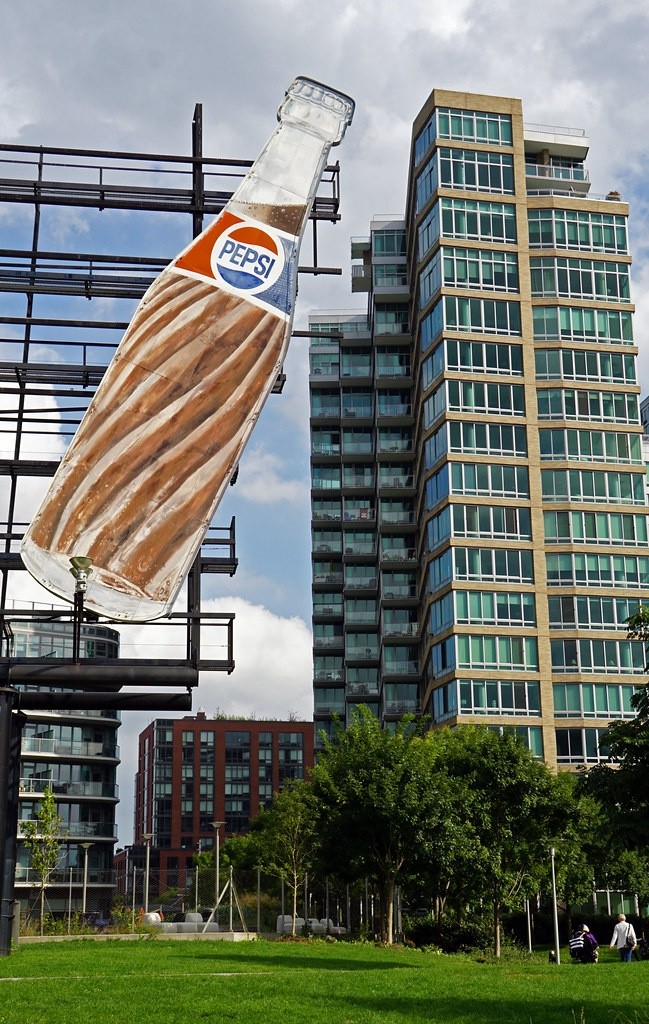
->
[16,76,355,622]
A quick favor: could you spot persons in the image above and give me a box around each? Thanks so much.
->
[609,914,637,962]
[570,924,599,964]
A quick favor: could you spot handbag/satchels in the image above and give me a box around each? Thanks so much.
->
[626,937,635,947]
[568,932,585,950]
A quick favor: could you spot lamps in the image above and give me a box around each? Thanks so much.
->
[69,556,93,665]
